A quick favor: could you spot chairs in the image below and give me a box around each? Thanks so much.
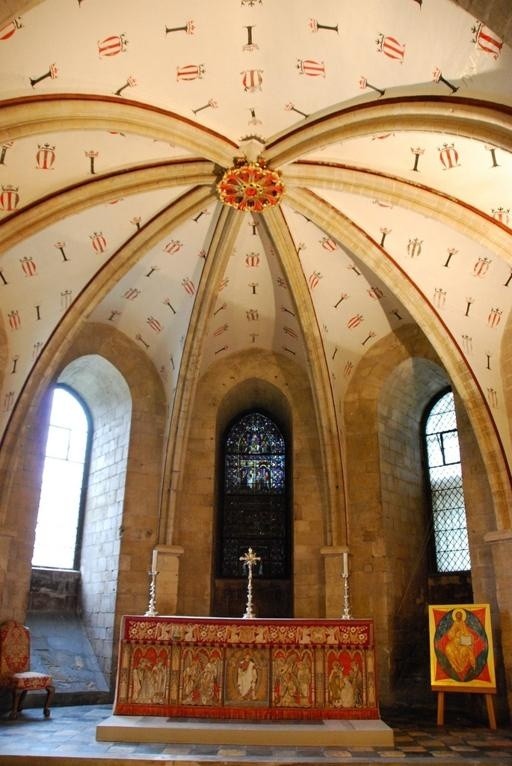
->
[0,619,55,719]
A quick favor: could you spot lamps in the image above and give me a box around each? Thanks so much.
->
[341,552,353,620]
[239,547,262,618]
[145,549,160,615]
